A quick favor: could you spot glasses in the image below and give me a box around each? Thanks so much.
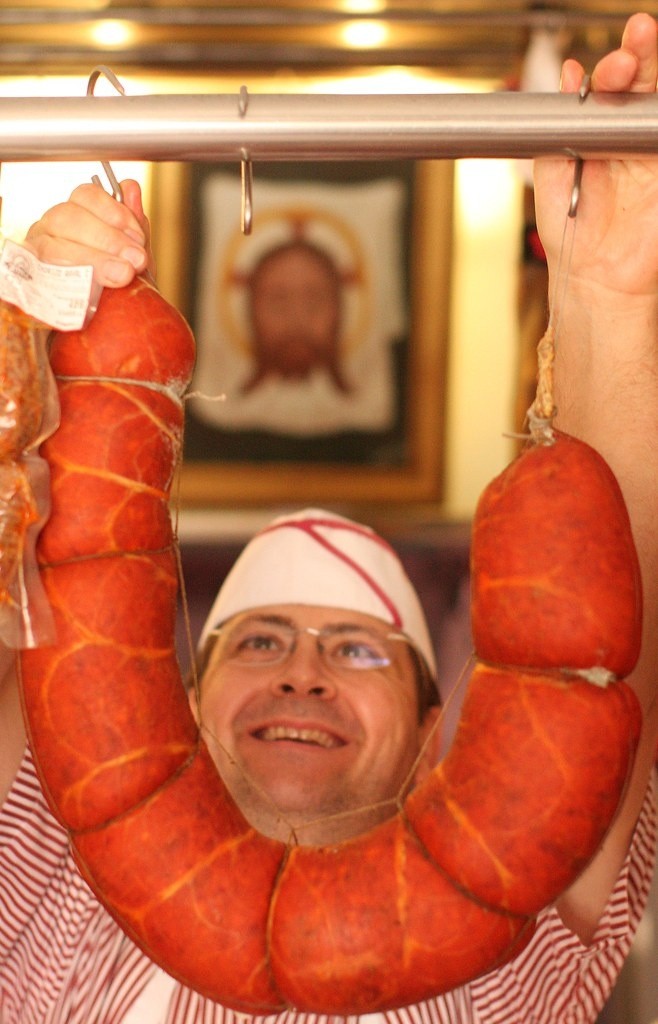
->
[203,613,431,704]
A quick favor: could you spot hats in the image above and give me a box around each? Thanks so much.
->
[195,505,440,685]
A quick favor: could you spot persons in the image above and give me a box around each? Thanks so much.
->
[0,5,658,1024]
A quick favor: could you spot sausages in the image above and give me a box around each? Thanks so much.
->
[16,278,643,1016]
